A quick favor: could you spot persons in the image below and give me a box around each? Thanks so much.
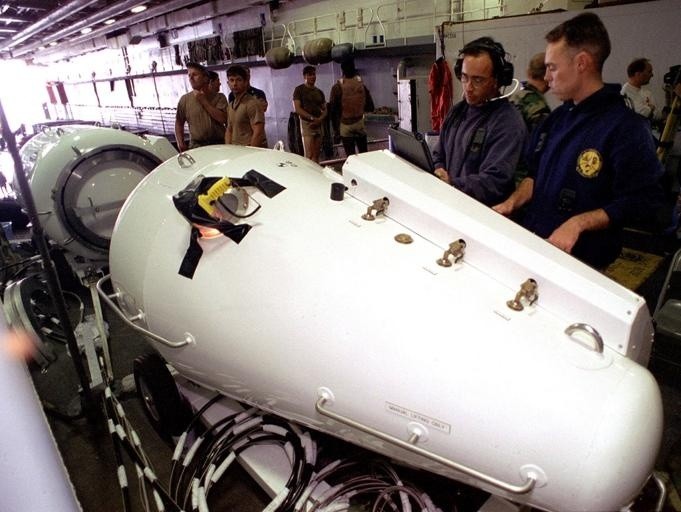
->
[508,51,552,194]
[206,70,221,92]
[224,65,265,147]
[228,65,267,109]
[293,65,327,164]
[434,37,529,206]
[175,61,227,149]
[617,59,656,121]
[492,13,665,270]
[329,57,375,156]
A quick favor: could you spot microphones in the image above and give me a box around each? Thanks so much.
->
[485,78,519,103]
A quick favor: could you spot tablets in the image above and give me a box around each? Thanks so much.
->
[387,125,435,172]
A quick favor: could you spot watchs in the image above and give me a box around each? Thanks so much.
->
[310,116,313,121]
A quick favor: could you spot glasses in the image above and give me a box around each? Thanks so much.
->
[458,73,490,86]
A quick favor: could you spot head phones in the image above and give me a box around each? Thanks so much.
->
[454,43,513,85]
